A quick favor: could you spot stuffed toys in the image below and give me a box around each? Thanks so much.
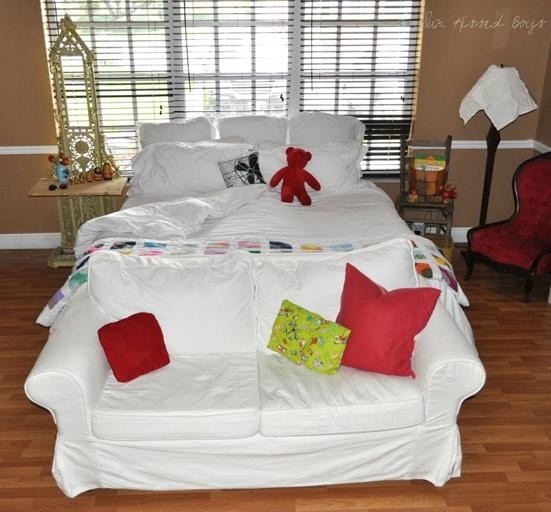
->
[269,147,323,206]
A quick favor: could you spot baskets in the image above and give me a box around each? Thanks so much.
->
[425,234,455,264]
[408,165,448,196]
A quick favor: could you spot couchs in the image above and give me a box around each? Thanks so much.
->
[21,237,487,498]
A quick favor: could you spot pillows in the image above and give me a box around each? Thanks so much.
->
[131,113,366,195]
[335,261,443,380]
[96,310,171,384]
[268,298,353,374]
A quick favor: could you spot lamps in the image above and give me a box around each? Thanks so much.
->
[459,64,539,264]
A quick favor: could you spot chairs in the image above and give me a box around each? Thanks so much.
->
[464,149,551,303]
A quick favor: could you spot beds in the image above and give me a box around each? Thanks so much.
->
[33,112,471,336]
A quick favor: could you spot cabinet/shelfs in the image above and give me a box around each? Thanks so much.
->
[394,135,456,249]
